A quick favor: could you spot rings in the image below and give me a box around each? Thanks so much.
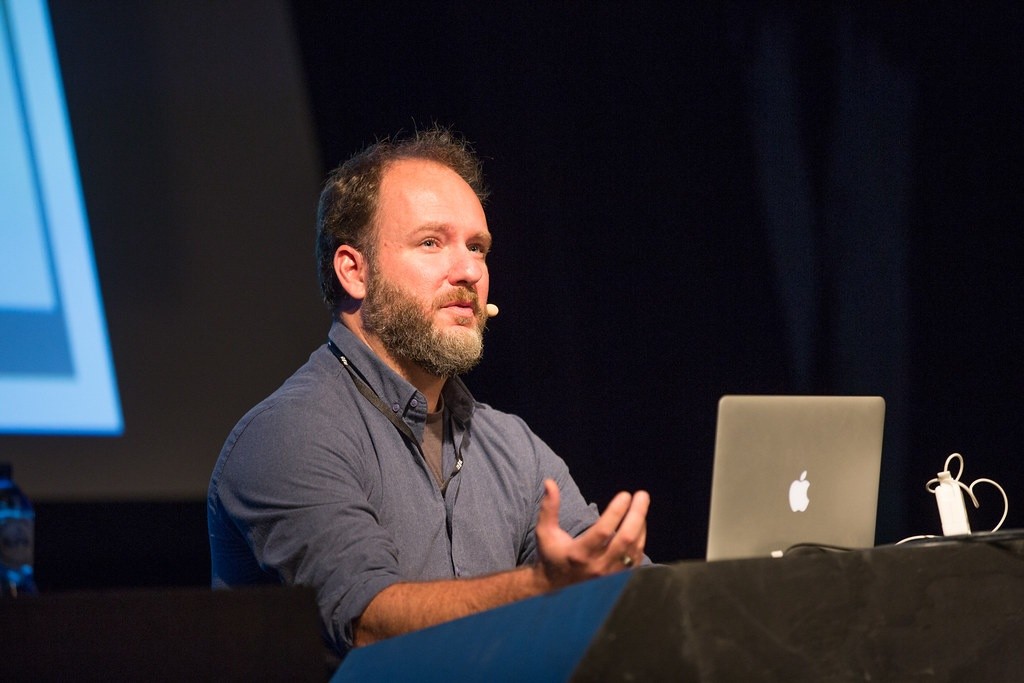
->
[621,555,632,567]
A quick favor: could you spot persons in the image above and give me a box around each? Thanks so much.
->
[205,126,651,682]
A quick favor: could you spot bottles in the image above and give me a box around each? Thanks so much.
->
[0,462,36,592]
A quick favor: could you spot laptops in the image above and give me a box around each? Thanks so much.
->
[706,395,885,560]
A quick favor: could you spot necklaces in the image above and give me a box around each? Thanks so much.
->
[426,393,444,422]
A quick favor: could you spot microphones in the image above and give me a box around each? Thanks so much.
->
[485,304,499,316]
